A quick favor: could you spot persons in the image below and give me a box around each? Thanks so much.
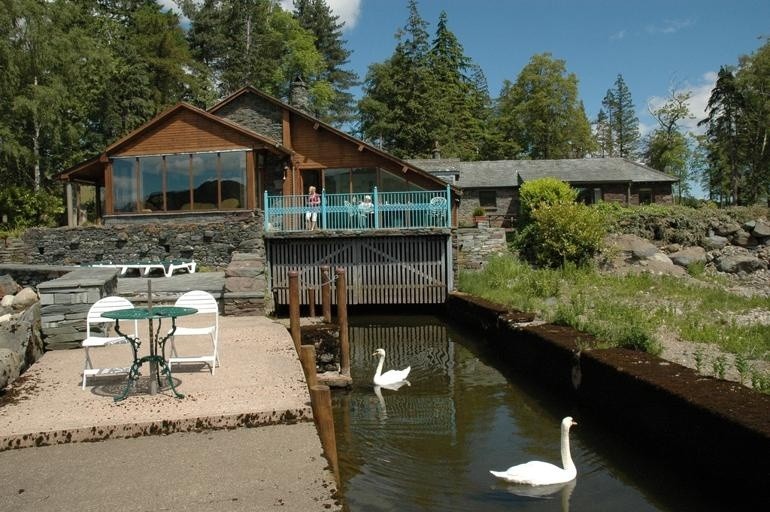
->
[305,186,320,230]
[358,195,374,213]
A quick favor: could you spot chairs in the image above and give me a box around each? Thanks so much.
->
[425,197,446,227]
[81,296,138,389]
[344,201,367,229]
[167,290,219,376]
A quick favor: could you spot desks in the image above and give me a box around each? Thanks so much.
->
[101,307,198,401]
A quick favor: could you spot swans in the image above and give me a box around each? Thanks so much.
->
[488,416,578,486]
[372,348,411,386]
